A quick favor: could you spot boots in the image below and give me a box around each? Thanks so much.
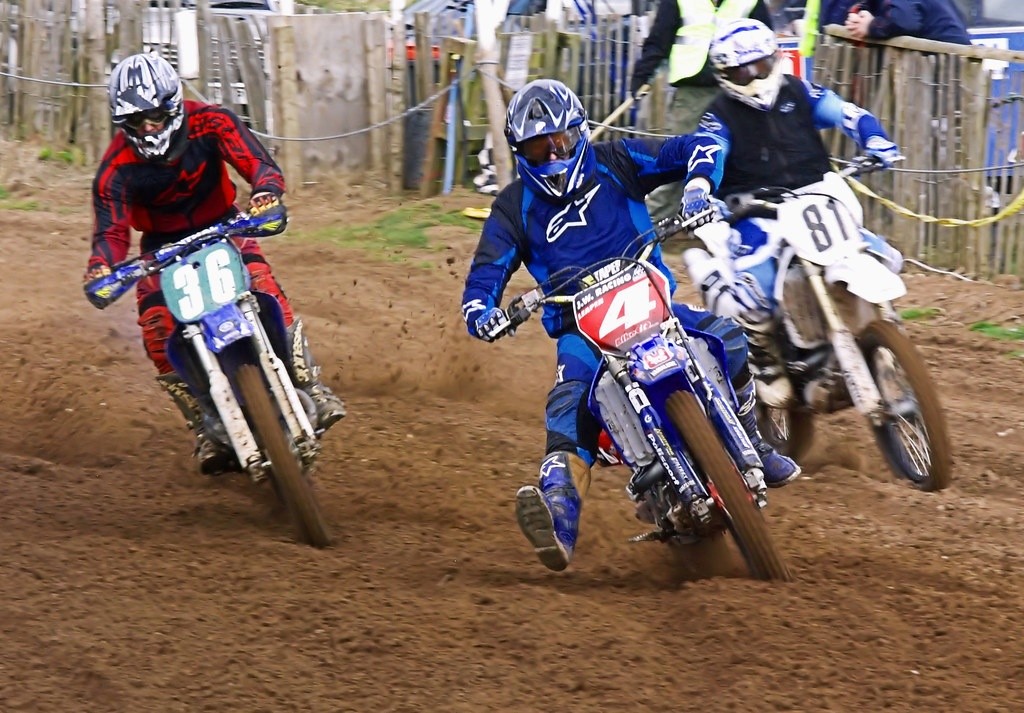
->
[515,452,591,572]
[734,313,793,409]
[732,372,801,488]
[156,371,224,475]
[285,315,347,427]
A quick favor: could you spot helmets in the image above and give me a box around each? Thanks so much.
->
[107,53,185,160]
[505,77,598,202]
[709,15,785,112]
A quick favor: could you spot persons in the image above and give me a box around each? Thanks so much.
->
[630,0,980,226]
[83,53,347,474]
[685,18,915,415]
[461,79,802,572]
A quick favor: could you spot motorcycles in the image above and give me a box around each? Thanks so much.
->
[711,148,954,493]
[84,203,335,551]
[485,194,797,588]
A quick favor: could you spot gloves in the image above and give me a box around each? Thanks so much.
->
[475,308,514,342]
[865,136,899,170]
[682,189,714,230]
[248,192,286,238]
[82,264,117,312]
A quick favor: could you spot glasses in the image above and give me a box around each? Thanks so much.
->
[519,125,582,166]
[121,107,168,130]
[719,54,776,87]
